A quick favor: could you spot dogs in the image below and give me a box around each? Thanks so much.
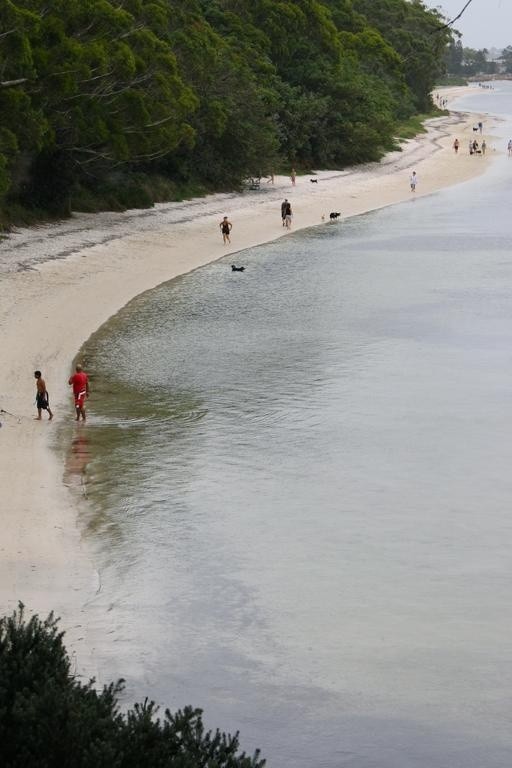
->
[310,179,317,184]
[231,265,245,272]
[473,128,478,132]
[330,213,340,221]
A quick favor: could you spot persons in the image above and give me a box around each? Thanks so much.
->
[410,170,417,192]
[281,199,288,227]
[284,203,293,230]
[507,139,512,155]
[469,140,473,154]
[473,140,478,156]
[291,167,296,185]
[453,138,459,155]
[481,140,486,155]
[69,363,90,423]
[220,216,234,244]
[33,371,55,420]
